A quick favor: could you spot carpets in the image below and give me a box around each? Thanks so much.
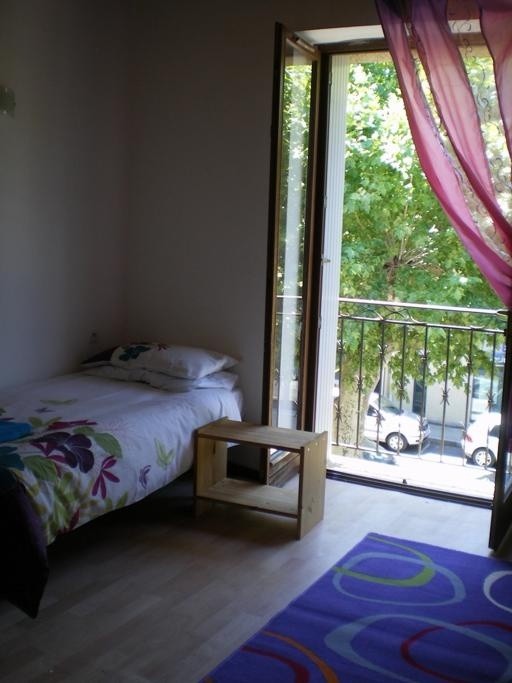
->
[197,529,511,682]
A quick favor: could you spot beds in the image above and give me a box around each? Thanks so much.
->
[0,369,245,622]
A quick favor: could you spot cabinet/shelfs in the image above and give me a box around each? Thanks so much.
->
[193,415,328,540]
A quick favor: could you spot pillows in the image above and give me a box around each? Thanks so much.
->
[80,337,242,392]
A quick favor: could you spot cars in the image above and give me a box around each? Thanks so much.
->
[460,411,503,469]
[333,389,430,452]
[273,365,341,409]
